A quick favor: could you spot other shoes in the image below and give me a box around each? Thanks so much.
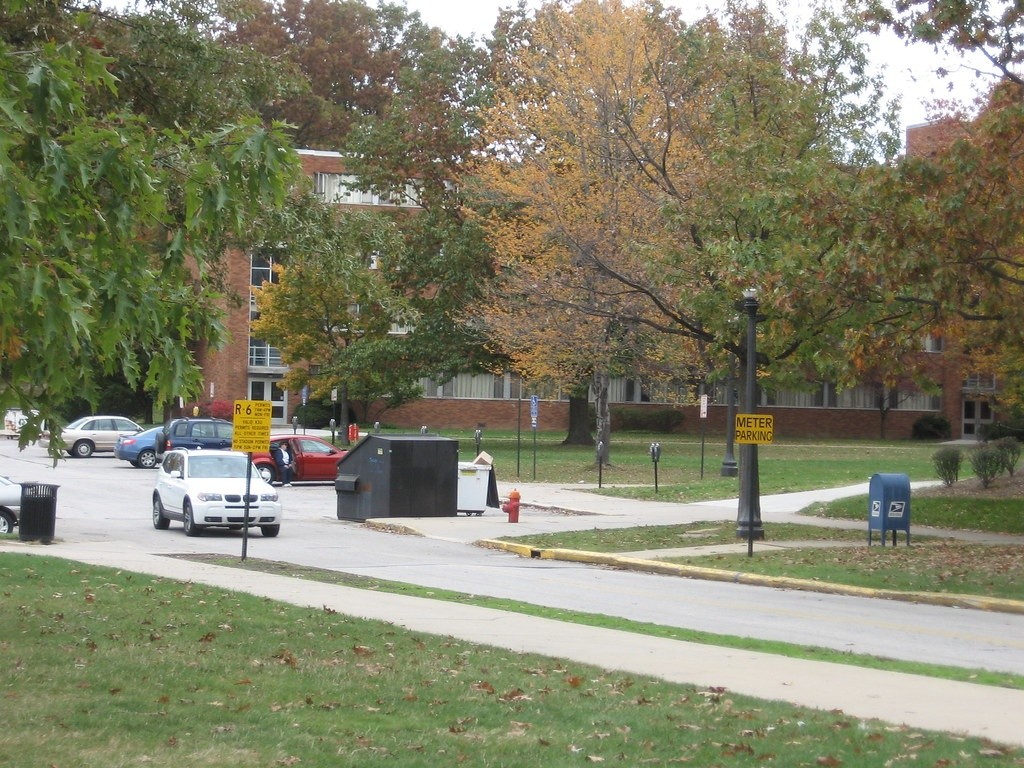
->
[284,483,292,486]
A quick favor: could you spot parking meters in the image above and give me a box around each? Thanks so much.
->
[473,429,482,456]
[291,416,298,435]
[649,442,661,495]
[596,440,606,490]
[419,426,428,434]
[330,418,337,445]
[373,421,380,434]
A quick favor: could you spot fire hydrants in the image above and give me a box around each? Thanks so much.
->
[501,487,522,524]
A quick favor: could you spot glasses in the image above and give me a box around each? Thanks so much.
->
[282,444,288,446]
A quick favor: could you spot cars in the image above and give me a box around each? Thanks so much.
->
[38,414,145,458]
[113,424,206,469]
[223,434,350,486]
[0,473,34,535]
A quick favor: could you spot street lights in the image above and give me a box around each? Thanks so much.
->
[737,285,768,540]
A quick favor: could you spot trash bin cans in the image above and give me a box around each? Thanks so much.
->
[19,482,61,545]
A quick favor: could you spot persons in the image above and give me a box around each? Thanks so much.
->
[273,442,293,487]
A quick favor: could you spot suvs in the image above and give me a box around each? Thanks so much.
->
[154,413,233,463]
[150,446,282,537]
